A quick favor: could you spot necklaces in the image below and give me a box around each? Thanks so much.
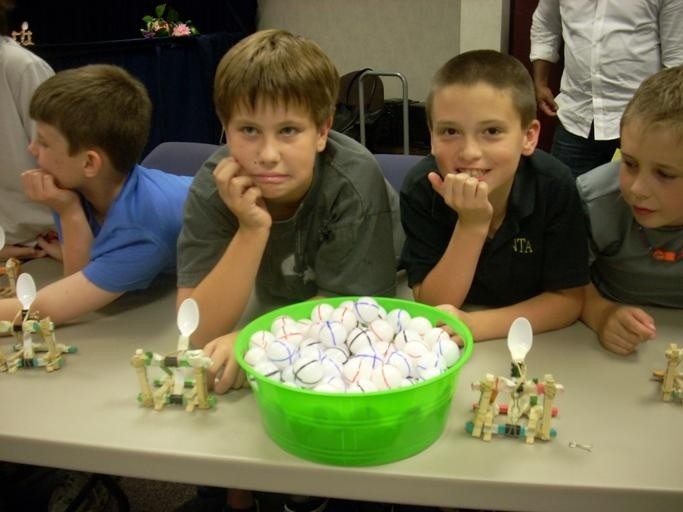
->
[634,219,683,263]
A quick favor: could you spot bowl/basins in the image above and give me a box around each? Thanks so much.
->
[234,294,473,466]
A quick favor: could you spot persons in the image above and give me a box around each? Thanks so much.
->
[0,64,195,327]
[400,50,589,345]
[0,1,63,261]
[576,64,683,356]
[174,29,407,395]
[530,1,683,180]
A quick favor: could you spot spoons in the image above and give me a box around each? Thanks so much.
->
[507,316,533,423]
[16,272,36,356]
[172,297,200,395]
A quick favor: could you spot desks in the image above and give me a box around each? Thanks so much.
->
[1,256,681,512]
